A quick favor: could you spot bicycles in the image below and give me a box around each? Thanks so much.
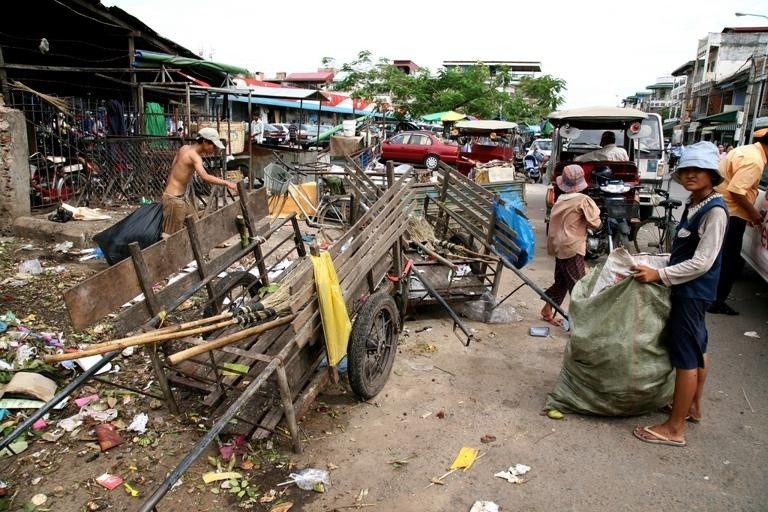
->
[633,186,684,263]
[258,148,380,242]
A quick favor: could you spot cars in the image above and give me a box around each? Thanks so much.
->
[250,122,335,155]
[371,131,461,171]
[524,137,557,172]
[738,163,768,293]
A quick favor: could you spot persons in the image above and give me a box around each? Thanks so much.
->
[251,111,264,144]
[573,132,629,163]
[629,140,729,443]
[717,144,724,154]
[707,132,767,316]
[540,165,602,318]
[723,145,733,156]
[517,133,523,153]
[160,127,237,234]
[288,120,297,148]
[667,142,684,172]
[398,126,404,132]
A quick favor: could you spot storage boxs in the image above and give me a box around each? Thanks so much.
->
[197,121,245,155]
[330,136,364,165]
[474,167,513,183]
[206,171,242,196]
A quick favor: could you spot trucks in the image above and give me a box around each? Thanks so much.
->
[554,113,665,222]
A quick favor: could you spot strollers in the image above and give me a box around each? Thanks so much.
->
[521,154,544,184]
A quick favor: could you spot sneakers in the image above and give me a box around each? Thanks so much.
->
[704,299,741,317]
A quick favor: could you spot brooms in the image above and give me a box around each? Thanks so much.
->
[405,209,501,272]
[41,256,325,366]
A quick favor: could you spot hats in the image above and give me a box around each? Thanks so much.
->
[555,163,588,193]
[670,141,726,187]
[195,128,225,149]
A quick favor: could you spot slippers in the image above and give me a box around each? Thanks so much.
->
[542,312,565,326]
[667,404,700,422]
[633,425,686,447]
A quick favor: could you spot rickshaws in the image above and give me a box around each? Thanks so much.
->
[341,151,576,344]
[0,179,420,508]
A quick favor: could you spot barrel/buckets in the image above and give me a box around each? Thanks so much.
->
[343,120,357,137]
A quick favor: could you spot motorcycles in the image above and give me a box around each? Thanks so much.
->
[453,119,518,186]
[543,105,648,260]
[22,107,174,210]
